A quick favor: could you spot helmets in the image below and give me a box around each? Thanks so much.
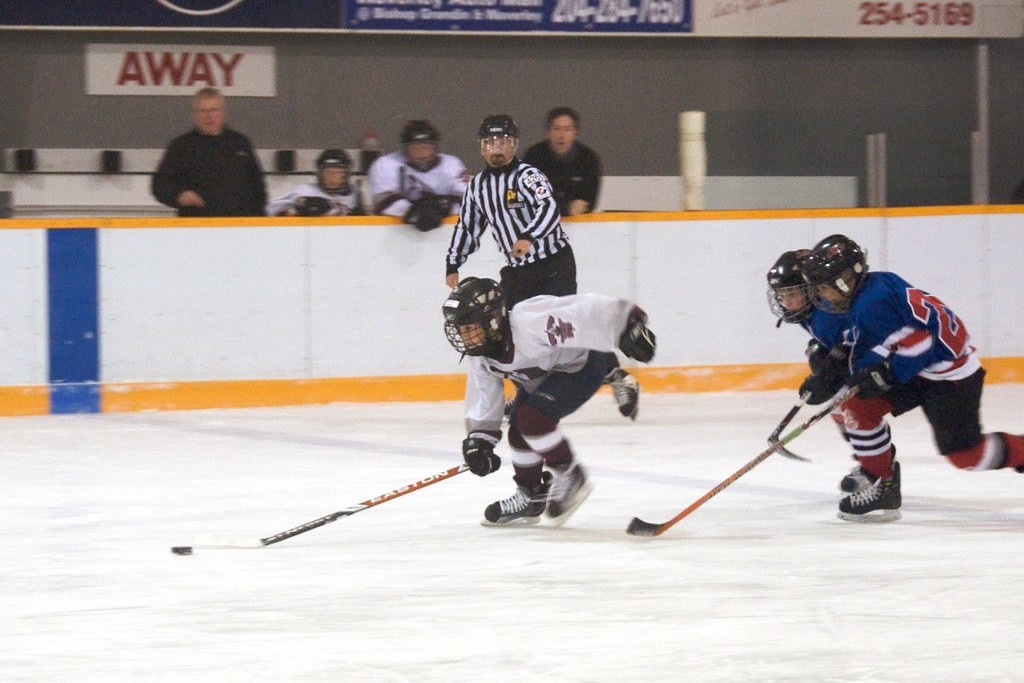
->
[316,149,352,168]
[477,114,519,169]
[442,277,508,356]
[766,249,813,324]
[802,234,868,315]
[399,120,439,143]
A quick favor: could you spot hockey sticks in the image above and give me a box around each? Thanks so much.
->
[625,381,871,538]
[195,463,470,548]
[765,390,819,465]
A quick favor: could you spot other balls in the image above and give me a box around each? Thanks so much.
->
[171,546,193,554]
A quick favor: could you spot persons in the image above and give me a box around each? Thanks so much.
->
[522,107,600,217]
[766,234,1024,515]
[368,120,473,231]
[442,277,656,524]
[446,114,577,415]
[152,87,269,216]
[262,149,365,216]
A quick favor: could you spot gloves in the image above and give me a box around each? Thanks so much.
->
[462,430,502,476]
[848,365,897,400]
[297,196,333,216]
[815,344,849,376]
[406,194,449,232]
[800,371,846,404]
[618,319,656,363]
[805,338,829,372]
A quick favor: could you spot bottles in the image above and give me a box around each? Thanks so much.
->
[361,133,378,149]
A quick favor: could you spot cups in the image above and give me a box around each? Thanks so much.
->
[16,150,33,170]
[102,151,118,173]
[0,191,12,218]
[277,150,294,172]
[360,151,379,175]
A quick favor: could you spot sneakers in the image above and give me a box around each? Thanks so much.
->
[840,444,896,492]
[547,462,594,527]
[480,476,548,527]
[837,463,902,524]
[604,369,639,420]
[503,398,516,419]
